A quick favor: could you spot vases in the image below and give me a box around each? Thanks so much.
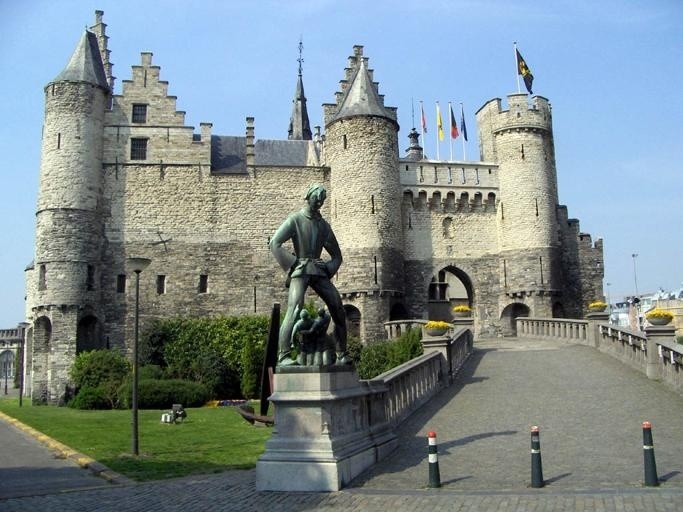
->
[648,317,671,325]
[423,328,449,336]
[451,311,472,317]
[590,307,605,312]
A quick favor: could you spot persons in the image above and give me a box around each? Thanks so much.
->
[291,309,315,366]
[269,186,354,366]
[299,307,334,366]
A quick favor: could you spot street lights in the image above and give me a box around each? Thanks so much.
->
[124,257,152,456]
[18,321,31,407]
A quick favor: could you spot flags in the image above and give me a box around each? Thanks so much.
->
[516,48,533,95]
[450,107,459,139]
[437,106,444,141]
[460,109,468,141]
[421,104,428,133]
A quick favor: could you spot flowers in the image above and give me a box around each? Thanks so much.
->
[424,320,448,330]
[451,305,470,312]
[645,308,673,320]
[587,302,608,308]
